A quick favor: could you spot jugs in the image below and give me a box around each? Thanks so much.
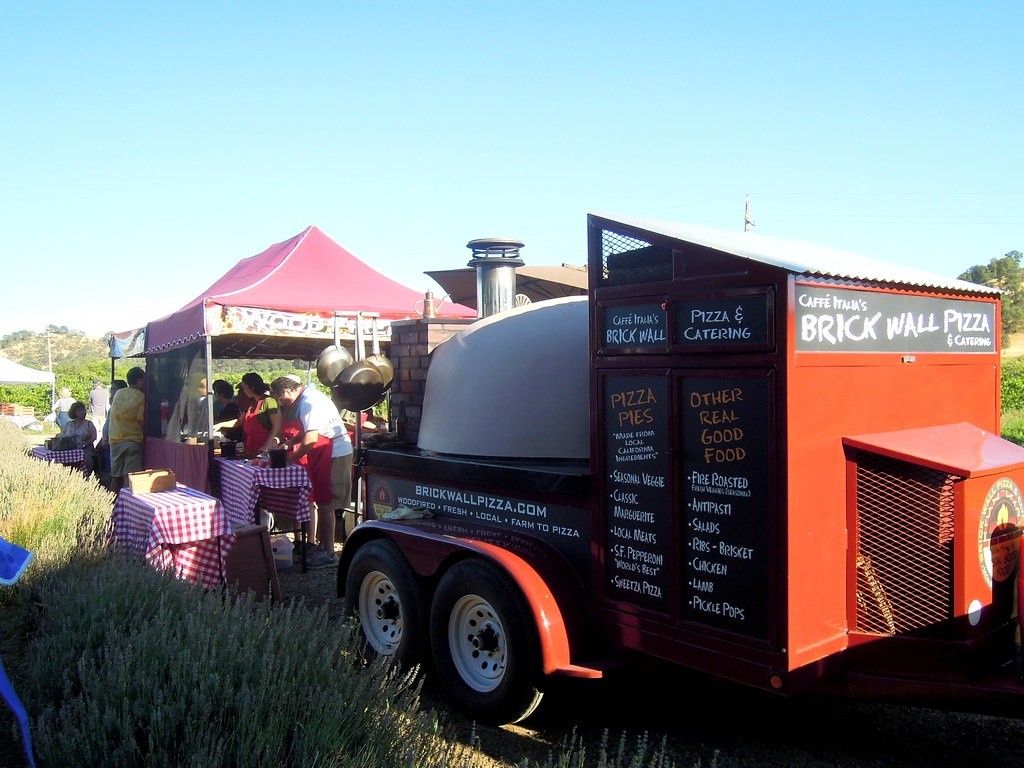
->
[414,288,451,318]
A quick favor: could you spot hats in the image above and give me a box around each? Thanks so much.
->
[91,379,103,386]
[58,387,72,399]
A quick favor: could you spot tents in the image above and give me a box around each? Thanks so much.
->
[107,225,478,496]
[0,356,56,430]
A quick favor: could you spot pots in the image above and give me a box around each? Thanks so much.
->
[315,315,395,413]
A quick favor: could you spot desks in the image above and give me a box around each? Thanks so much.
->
[226,522,282,608]
[32,446,87,478]
[106,482,236,596]
[214,453,311,574]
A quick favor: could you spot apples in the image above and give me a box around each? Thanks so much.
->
[250,459,267,468]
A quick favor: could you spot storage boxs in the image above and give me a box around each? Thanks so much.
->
[128,468,176,494]
[269,535,294,571]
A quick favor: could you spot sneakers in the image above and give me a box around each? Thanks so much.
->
[305,543,340,570]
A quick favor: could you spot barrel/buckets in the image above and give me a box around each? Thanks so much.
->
[220,442,237,458]
[268,449,287,468]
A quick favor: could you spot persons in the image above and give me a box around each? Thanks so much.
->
[270,376,353,568]
[212,379,241,424]
[109,366,145,494]
[51,387,77,433]
[89,380,109,441]
[102,379,128,479]
[61,402,97,475]
[232,373,279,459]
